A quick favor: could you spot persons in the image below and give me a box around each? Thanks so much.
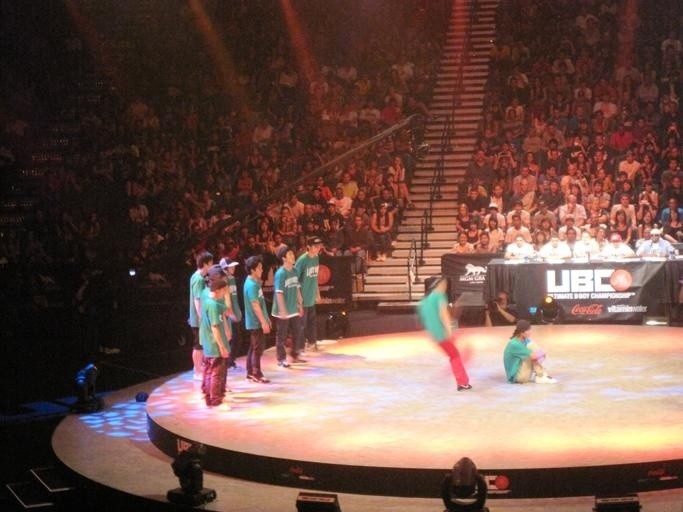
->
[188,236,325,410]
[416,277,473,392]
[504,319,558,385]
[0,0,443,295]
[484,292,517,328]
[456,0,682,259]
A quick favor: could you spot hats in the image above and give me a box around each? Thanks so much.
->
[207,257,239,274]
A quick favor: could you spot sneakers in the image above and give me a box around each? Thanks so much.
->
[535,372,558,385]
[193,344,324,411]
[457,384,471,391]
